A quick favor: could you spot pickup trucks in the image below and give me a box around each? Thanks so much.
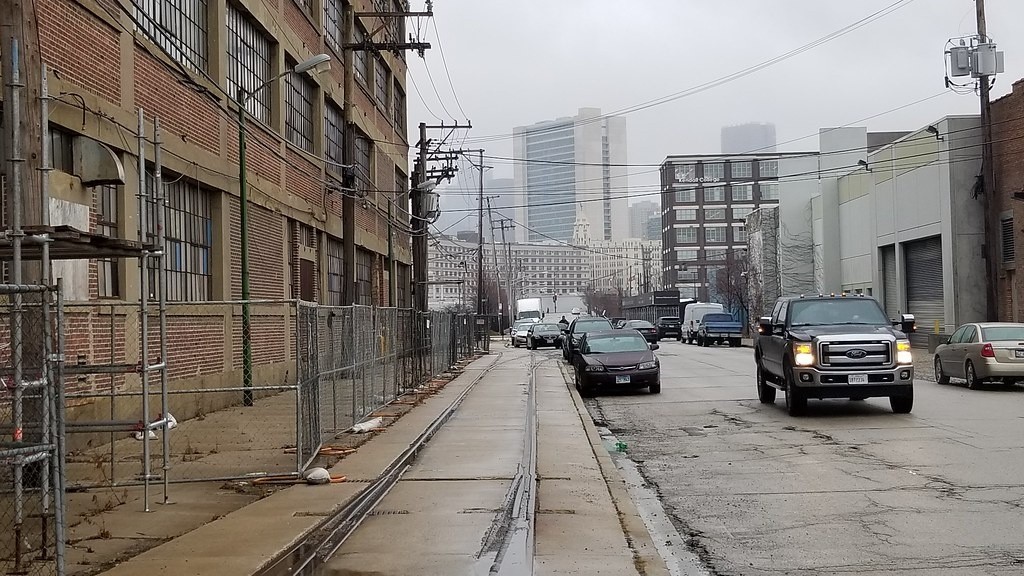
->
[697,311,743,348]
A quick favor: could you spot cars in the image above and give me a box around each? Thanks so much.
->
[932,323,1024,389]
[572,328,660,398]
[622,319,658,345]
[513,323,538,347]
[571,308,580,315]
[510,320,535,344]
[525,323,561,351]
[655,316,681,342]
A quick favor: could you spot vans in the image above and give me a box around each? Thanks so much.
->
[681,304,724,343]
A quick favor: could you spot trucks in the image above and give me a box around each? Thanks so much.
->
[514,297,542,323]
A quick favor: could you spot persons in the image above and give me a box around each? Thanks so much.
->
[559,315,569,349]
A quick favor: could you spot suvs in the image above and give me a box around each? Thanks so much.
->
[752,290,915,418]
[562,316,615,365]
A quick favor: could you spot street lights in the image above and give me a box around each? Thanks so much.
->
[236,53,333,404]
[387,180,436,308]
